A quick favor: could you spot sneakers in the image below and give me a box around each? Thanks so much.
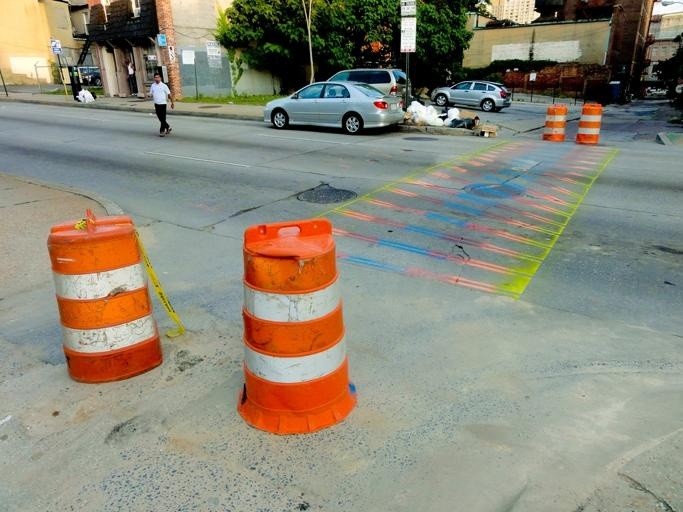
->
[159,127,172,136]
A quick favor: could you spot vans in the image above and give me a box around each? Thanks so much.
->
[324,67,412,109]
[430,79,512,113]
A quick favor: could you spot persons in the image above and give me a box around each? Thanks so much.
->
[126,61,138,96]
[146,72,174,137]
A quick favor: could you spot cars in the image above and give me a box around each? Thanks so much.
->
[81,69,103,87]
[263,80,405,136]
